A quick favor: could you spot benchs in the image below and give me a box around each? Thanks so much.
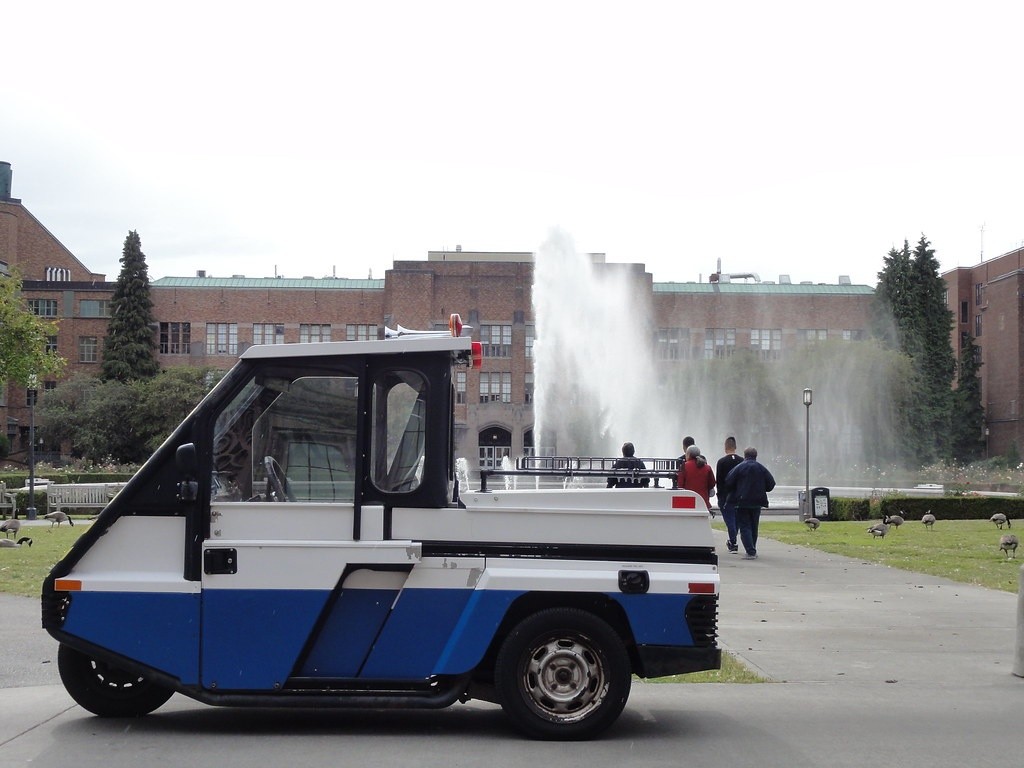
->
[47,483,109,511]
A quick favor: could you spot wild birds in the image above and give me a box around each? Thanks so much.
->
[0,506,33,549]
[864,510,908,540]
[41,510,75,529]
[988,512,1011,530]
[804,517,821,532]
[999,532,1018,558]
[920,510,937,531]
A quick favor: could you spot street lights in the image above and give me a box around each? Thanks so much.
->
[24,370,39,520]
[802,388,812,521]
[985,428,989,458]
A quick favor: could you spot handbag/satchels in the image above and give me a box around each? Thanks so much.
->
[709,488,715,497]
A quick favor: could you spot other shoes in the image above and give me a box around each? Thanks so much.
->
[745,551,756,560]
[725,540,738,554]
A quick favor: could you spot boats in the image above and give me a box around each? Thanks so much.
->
[20,478,51,490]
[914,483,943,490]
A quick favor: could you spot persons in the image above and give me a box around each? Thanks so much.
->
[672,437,707,487]
[678,445,716,510]
[606,442,650,488]
[725,447,776,559]
[716,437,744,554]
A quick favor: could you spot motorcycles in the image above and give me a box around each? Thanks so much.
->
[40,315,721,743]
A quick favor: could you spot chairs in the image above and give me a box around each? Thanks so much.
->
[0,483,17,519]
